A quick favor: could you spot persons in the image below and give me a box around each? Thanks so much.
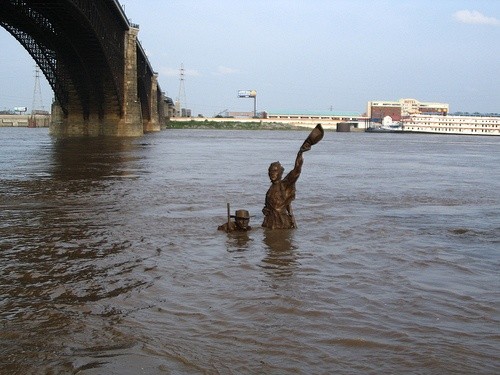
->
[260,123,324,230]
[218,202,254,233]
